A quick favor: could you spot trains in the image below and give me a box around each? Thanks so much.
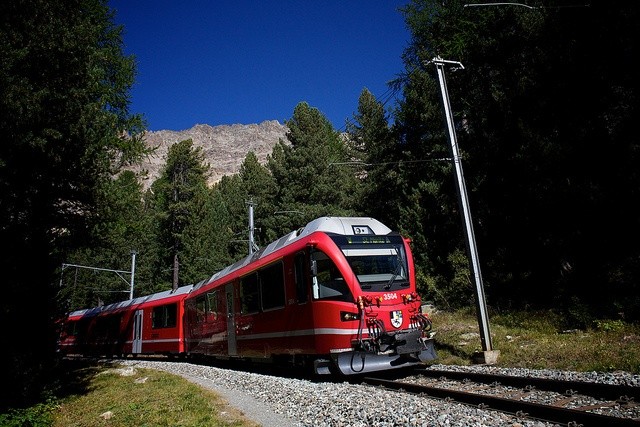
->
[54,215,438,383]
